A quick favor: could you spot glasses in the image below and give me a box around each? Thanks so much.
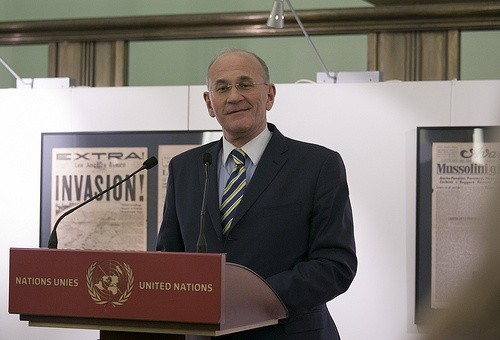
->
[208,80,268,96]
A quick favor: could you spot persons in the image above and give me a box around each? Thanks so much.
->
[155,49,358,340]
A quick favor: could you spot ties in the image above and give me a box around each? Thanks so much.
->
[220,148,247,239]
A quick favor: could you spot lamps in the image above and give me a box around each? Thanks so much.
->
[266,0,337,80]
